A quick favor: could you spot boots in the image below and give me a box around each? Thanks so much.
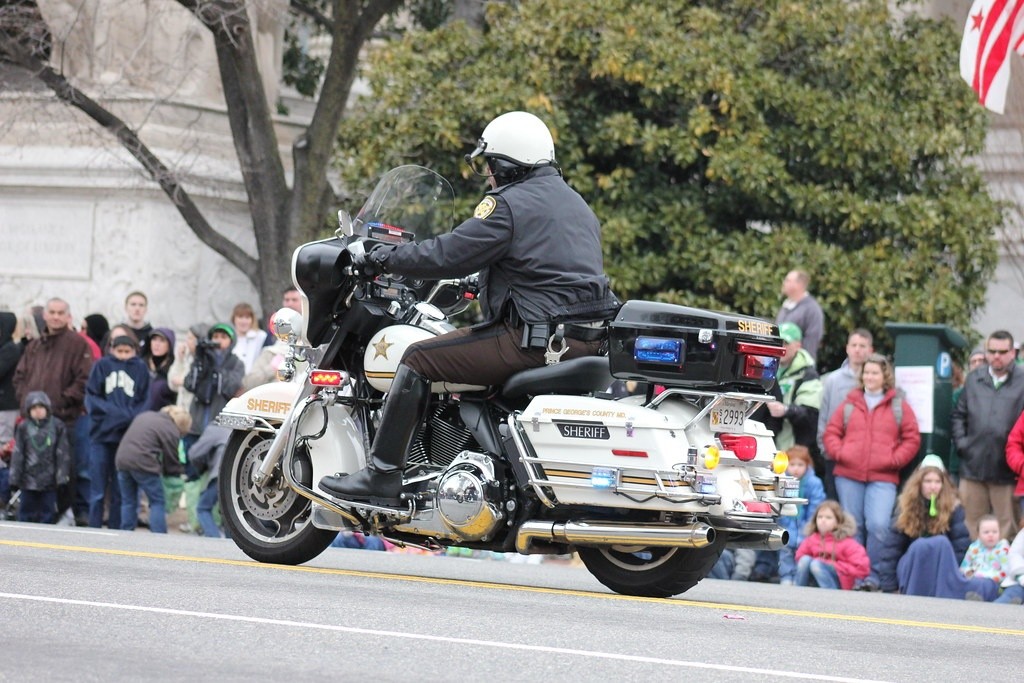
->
[318,362,432,507]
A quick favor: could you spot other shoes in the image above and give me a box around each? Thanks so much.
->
[76,511,89,525]
[862,584,874,590]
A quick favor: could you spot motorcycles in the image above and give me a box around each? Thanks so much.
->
[215,163,811,600]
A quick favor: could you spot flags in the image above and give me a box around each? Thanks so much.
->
[958,0,1024,114]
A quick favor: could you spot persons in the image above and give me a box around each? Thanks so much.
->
[705,323,1024,605]
[0,287,304,538]
[331,530,585,567]
[775,270,824,360]
[318,112,623,508]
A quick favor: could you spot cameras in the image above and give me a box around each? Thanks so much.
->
[201,341,220,350]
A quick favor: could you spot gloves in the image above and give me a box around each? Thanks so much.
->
[347,240,384,276]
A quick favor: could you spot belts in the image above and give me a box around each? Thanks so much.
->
[550,318,609,341]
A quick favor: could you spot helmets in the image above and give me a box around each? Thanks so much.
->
[470,111,556,167]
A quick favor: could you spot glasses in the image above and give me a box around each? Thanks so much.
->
[82,326,88,330]
[988,349,1013,354]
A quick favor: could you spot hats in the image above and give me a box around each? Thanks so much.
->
[209,325,234,341]
[152,331,167,339]
[920,455,944,472]
[778,323,802,343]
[114,336,133,348]
